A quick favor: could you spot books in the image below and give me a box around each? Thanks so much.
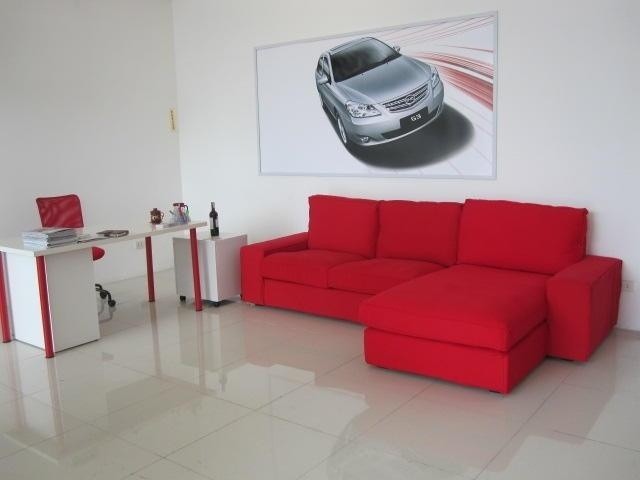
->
[21,226,77,248]
[97,230,129,238]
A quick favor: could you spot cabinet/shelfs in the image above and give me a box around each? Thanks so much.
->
[171,230,249,308]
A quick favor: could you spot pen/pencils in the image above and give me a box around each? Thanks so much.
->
[170,211,175,216]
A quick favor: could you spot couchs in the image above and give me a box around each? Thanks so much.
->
[238,193,625,394]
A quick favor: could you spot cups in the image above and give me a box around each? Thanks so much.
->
[150,208,164,224]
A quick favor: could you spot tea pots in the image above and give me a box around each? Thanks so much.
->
[167,203,190,226]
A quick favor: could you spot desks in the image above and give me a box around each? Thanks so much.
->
[1,219,209,360]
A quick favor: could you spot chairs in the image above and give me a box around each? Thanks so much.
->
[35,193,119,312]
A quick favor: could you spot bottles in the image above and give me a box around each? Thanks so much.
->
[208,201,219,236]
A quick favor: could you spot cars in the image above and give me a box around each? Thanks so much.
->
[314,36,444,150]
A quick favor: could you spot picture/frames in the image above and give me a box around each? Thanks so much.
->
[252,6,500,183]
